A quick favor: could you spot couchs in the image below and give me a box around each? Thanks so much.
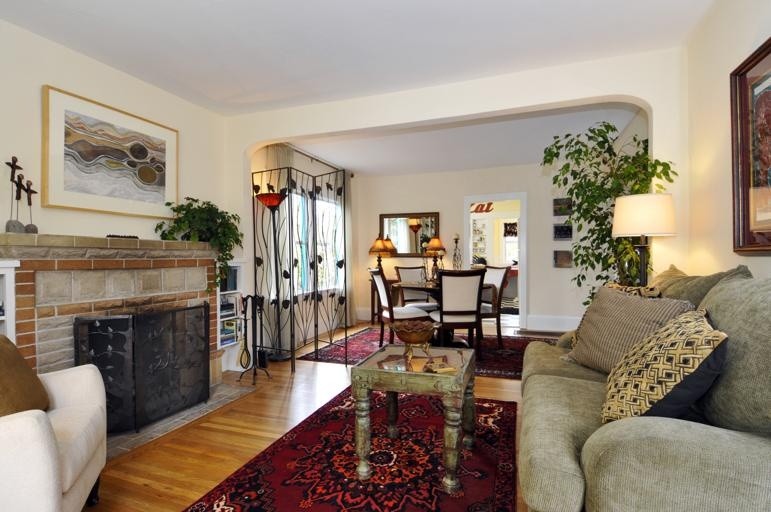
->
[519,265,771,512]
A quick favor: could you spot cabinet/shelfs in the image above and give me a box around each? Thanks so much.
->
[219,264,240,349]
[0,260,21,343]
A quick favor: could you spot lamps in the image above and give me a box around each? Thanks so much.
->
[611,193,678,286]
[408,218,422,252]
[427,233,445,278]
[368,233,389,267]
[384,235,397,253]
[256,192,292,362]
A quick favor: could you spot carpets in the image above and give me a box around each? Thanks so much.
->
[296,326,558,380]
[181,382,517,512]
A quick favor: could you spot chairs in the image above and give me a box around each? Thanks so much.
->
[1,332,107,512]
[368,266,512,362]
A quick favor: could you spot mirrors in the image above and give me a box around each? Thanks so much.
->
[380,211,439,258]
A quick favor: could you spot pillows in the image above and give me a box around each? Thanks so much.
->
[560,287,694,374]
[1,333,50,417]
[600,308,728,426]
[570,279,660,352]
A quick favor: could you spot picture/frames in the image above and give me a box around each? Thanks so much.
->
[730,38,771,257]
[41,84,180,221]
